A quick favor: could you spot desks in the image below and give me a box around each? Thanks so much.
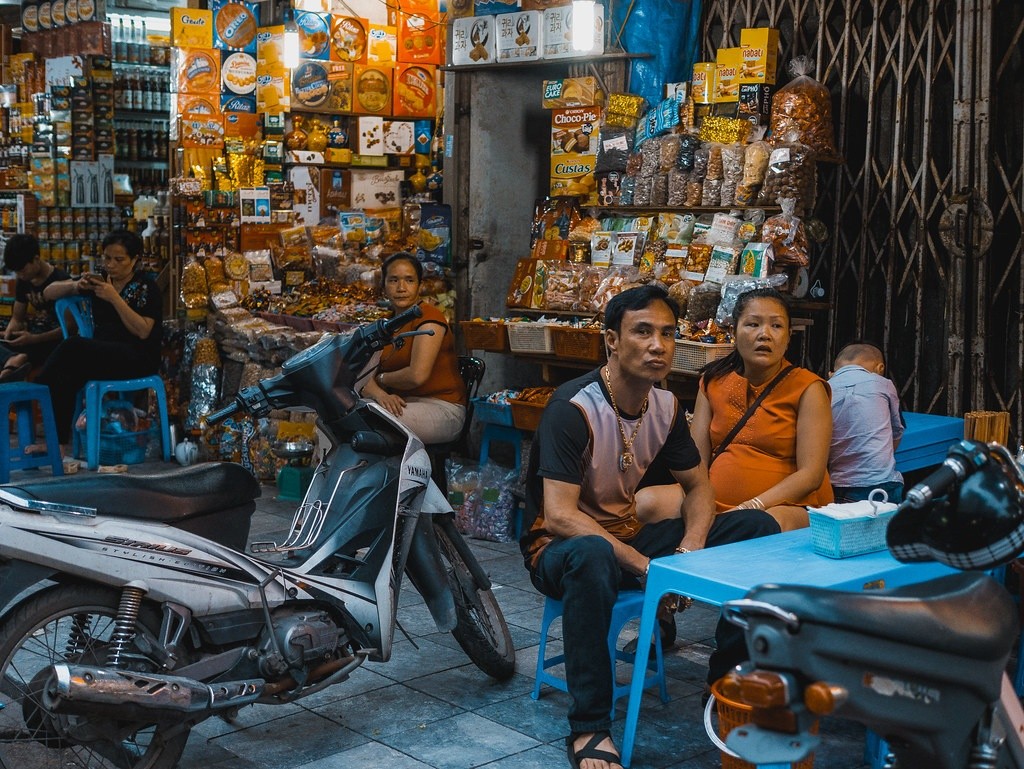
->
[892,412,965,473]
[622,526,1008,769]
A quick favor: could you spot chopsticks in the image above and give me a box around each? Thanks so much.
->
[965,412,1009,461]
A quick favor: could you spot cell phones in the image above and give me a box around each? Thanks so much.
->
[89,274,105,286]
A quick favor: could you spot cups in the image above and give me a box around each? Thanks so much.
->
[176,439,198,467]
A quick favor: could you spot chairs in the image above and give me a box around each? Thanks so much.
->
[55,294,172,472]
[426,357,485,499]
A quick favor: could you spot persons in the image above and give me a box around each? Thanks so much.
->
[296,253,470,531]
[520,286,782,769]
[24,228,165,458]
[826,341,904,507]
[623,287,835,657]
[0,235,83,384]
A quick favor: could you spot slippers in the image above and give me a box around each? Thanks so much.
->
[0,362,32,382]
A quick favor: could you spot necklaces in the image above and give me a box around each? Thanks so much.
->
[605,364,648,472]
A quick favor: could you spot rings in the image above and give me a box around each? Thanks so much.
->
[662,594,694,614]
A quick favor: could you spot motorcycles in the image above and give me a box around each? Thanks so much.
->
[0,303,516,769]
[716,438,1024,769]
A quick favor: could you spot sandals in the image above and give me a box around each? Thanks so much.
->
[565,730,622,769]
[702,672,718,712]
[623,616,676,657]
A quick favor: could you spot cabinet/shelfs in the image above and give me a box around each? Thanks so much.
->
[485,205,841,396]
[107,6,172,170]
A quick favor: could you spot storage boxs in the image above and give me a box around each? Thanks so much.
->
[662,25,781,135]
[452,0,608,64]
[809,508,899,560]
[460,321,735,431]
[164,1,445,292]
[504,74,606,305]
[22,22,112,57]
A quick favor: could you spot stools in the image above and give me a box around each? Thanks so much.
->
[530,590,671,719]
[482,426,531,546]
[0,380,63,484]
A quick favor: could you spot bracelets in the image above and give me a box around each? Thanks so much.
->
[674,547,690,554]
[635,556,652,584]
[737,498,765,511]
[377,373,384,387]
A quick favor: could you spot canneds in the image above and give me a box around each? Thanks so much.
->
[37,206,123,276]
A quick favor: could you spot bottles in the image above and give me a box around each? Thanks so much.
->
[0,194,18,231]
[0,145,28,187]
[410,160,441,193]
[285,115,348,151]
[112,166,170,211]
[106,16,150,65]
[127,216,169,260]
[332,172,342,190]
[114,68,171,114]
[113,121,170,161]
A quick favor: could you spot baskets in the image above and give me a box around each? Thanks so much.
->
[713,673,820,769]
[80,417,160,469]
[807,509,901,558]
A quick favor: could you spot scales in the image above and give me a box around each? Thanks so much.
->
[272,441,316,501]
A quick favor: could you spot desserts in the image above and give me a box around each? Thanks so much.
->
[551,129,595,196]
